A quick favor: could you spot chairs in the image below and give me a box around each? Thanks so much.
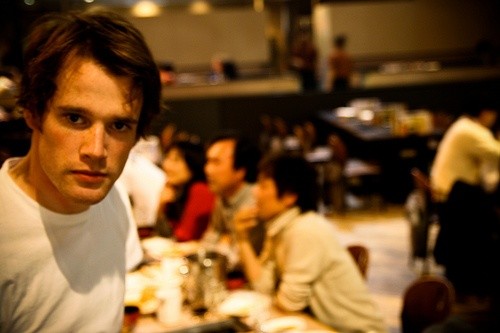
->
[408,170,447,274]
[403,277,455,333]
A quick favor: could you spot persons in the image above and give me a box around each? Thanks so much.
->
[0,7,162,333]
[0,31,500,333]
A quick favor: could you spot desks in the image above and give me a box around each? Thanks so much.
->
[119,114,443,333]
